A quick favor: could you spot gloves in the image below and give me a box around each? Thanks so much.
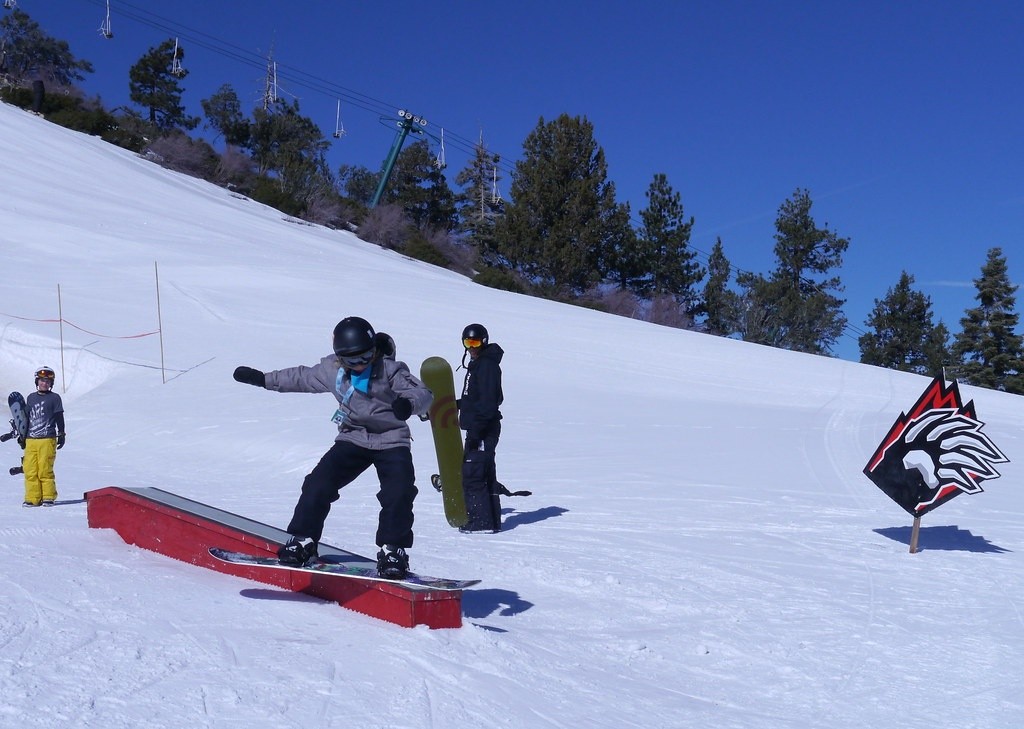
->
[234,367,266,389]
[17,436,26,450]
[391,397,413,421]
[468,435,482,451]
[56,433,66,450]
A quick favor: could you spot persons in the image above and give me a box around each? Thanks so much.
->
[455,324,505,533]
[22,366,66,507]
[233,316,435,580]
[335,416,342,421]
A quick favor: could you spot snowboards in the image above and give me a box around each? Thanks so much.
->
[8,391,29,437]
[207,546,482,590]
[418,356,468,527]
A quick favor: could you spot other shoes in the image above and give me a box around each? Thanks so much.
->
[21,500,55,508]
[457,522,501,533]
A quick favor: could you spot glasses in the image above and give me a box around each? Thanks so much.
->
[462,335,486,349]
[37,370,55,380]
[340,350,374,368]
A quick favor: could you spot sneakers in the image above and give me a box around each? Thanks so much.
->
[277,536,321,566]
[375,545,409,579]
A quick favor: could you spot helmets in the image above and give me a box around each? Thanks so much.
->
[34,366,55,386]
[461,323,489,350]
[333,316,378,371]
